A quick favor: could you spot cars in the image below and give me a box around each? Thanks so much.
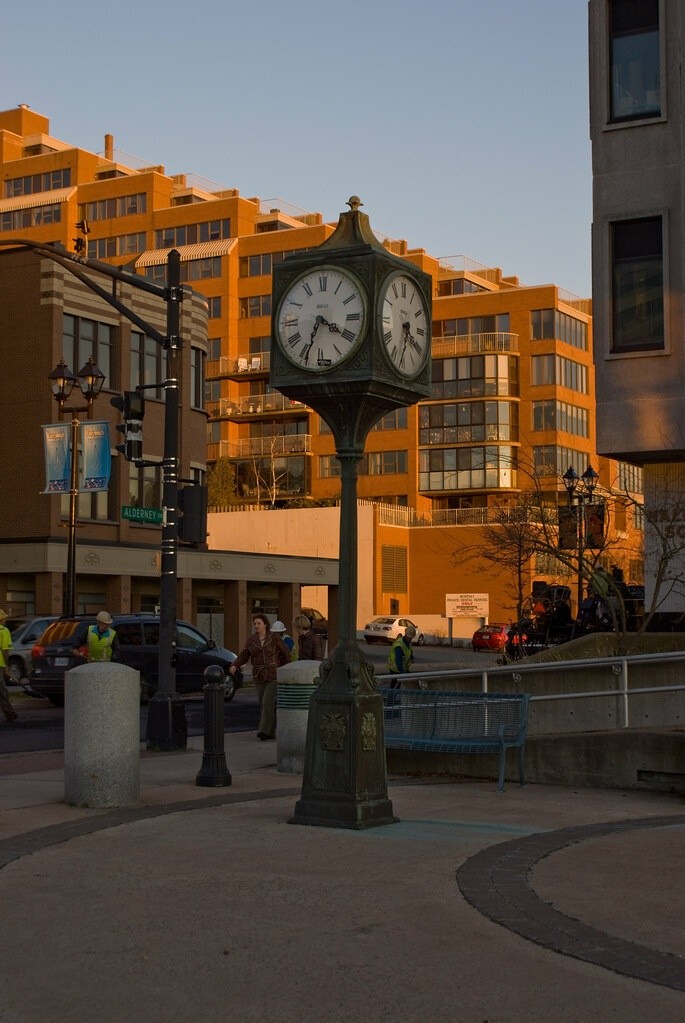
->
[471,622,527,654]
[364,616,426,647]
[301,606,329,640]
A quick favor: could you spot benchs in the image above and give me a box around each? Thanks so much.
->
[378,688,532,793]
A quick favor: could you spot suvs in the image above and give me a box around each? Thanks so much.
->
[19,611,245,707]
[2,614,62,686]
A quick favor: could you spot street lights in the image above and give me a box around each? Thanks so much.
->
[560,464,600,610]
[47,354,108,617]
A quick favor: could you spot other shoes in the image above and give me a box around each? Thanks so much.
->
[257,732,274,741]
[6,713,17,719]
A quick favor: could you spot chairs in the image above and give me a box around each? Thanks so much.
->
[237,357,261,373]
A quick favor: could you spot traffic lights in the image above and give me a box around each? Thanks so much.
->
[109,391,146,461]
[176,485,208,545]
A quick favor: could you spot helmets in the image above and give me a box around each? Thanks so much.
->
[405,626,416,638]
[270,621,287,632]
[96,611,113,624]
[0,609,8,620]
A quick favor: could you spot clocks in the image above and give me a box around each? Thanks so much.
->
[275,264,371,374]
[376,269,432,380]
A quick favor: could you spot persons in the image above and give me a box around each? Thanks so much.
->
[229,614,292,741]
[386,626,416,717]
[587,563,613,599]
[270,620,299,662]
[504,630,516,658]
[71,611,121,663]
[547,586,580,644]
[293,615,322,661]
[0,609,19,722]
